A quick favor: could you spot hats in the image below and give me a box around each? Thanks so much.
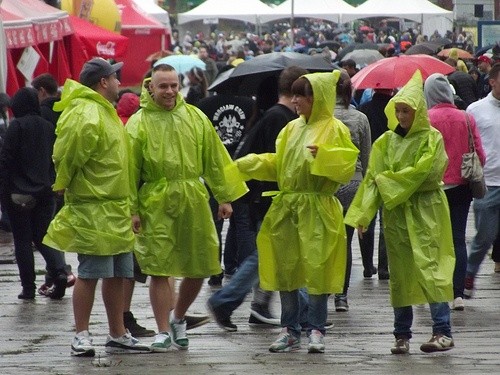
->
[478,55,492,65]
[79,56,124,85]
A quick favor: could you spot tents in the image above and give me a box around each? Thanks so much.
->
[0,0,454,129]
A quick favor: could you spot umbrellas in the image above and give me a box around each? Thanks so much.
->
[207,51,346,98]
[351,48,455,90]
[318,40,341,49]
[338,44,385,69]
[404,35,474,59]
[153,54,207,92]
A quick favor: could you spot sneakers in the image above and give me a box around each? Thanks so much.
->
[105,328,152,355]
[390,338,409,354]
[169,318,189,349]
[151,331,172,353]
[308,328,326,353]
[420,322,455,353]
[69,330,95,357]
[269,332,301,353]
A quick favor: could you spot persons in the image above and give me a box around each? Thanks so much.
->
[42,59,151,357]
[0,19,500,329]
[233,71,362,352]
[125,63,250,353]
[343,67,454,353]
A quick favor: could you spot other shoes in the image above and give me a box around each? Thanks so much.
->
[123,311,156,337]
[493,261,500,272]
[18,292,34,299]
[301,322,334,332]
[48,275,67,298]
[65,264,76,287]
[453,296,464,309]
[363,266,389,280]
[38,284,50,295]
[463,276,474,297]
[248,310,279,325]
[184,315,210,329]
[206,295,237,332]
[334,294,349,312]
[208,268,225,286]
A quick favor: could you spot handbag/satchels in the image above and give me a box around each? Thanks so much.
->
[461,151,486,199]
